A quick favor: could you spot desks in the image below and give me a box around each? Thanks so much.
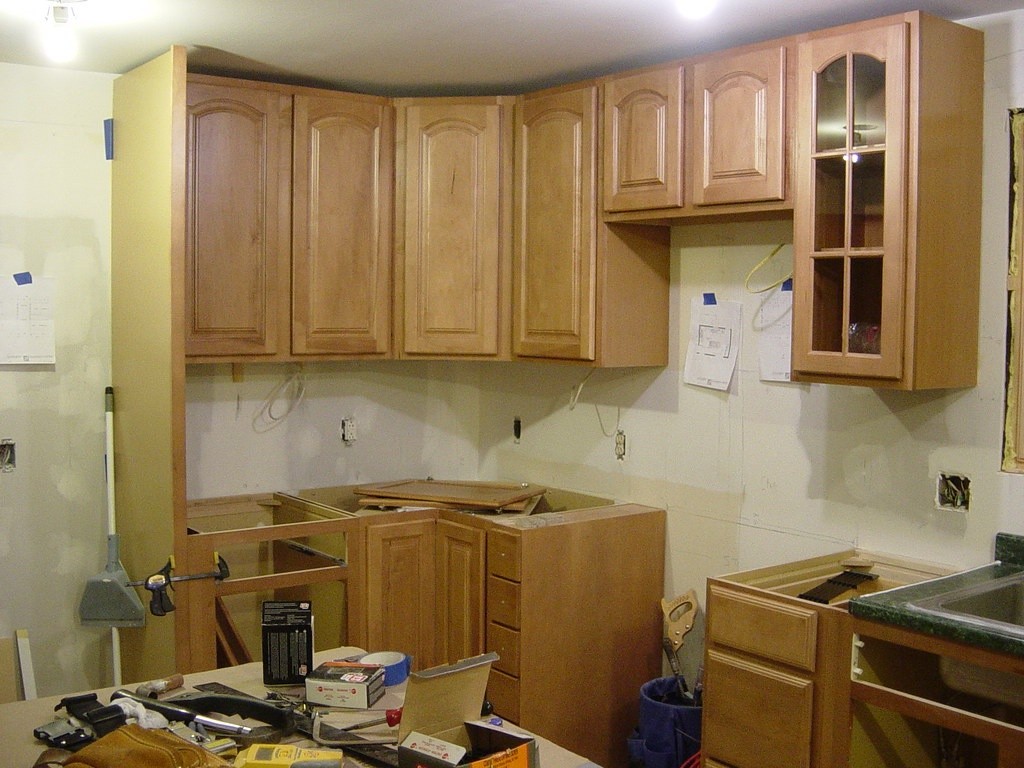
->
[0,647,606,768]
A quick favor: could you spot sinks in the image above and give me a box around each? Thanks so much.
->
[910,570,1023,640]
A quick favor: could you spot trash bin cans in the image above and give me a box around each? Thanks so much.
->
[626,677,702,768]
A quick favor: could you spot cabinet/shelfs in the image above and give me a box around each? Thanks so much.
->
[173,477,668,768]
[185,11,985,394]
[701,547,1024,768]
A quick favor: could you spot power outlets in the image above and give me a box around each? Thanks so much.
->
[614,434,625,456]
[343,418,357,441]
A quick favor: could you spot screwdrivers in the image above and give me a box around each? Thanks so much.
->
[340,706,404,731]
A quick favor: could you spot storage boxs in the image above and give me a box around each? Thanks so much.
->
[398,651,539,768]
[261,599,314,688]
[305,661,386,710]
